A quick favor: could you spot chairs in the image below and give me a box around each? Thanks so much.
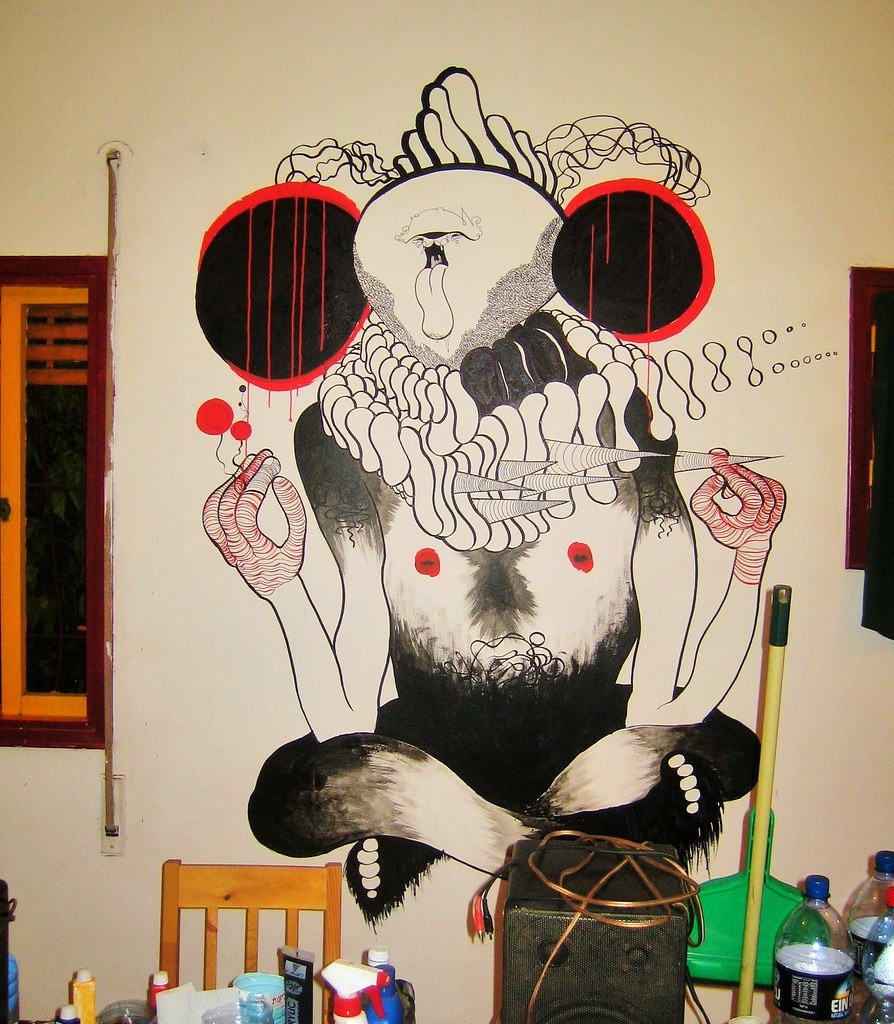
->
[160,859,341,1024]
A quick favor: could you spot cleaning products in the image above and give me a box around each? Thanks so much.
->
[319,957,390,1024]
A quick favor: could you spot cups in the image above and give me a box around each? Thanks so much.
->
[95,999,157,1024]
[202,1001,275,1024]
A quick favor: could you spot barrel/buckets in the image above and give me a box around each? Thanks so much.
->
[232,972,285,1024]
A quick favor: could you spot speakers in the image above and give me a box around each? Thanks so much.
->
[501,839,690,1024]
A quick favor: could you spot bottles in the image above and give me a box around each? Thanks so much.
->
[364,965,404,1024]
[6,951,19,1024]
[772,874,856,1024]
[362,947,394,1010]
[841,851,894,1024]
[56,1004,80,1024]
[72,969,95,1024]
[150,971,170,1008]
[859,886,894,1024]
